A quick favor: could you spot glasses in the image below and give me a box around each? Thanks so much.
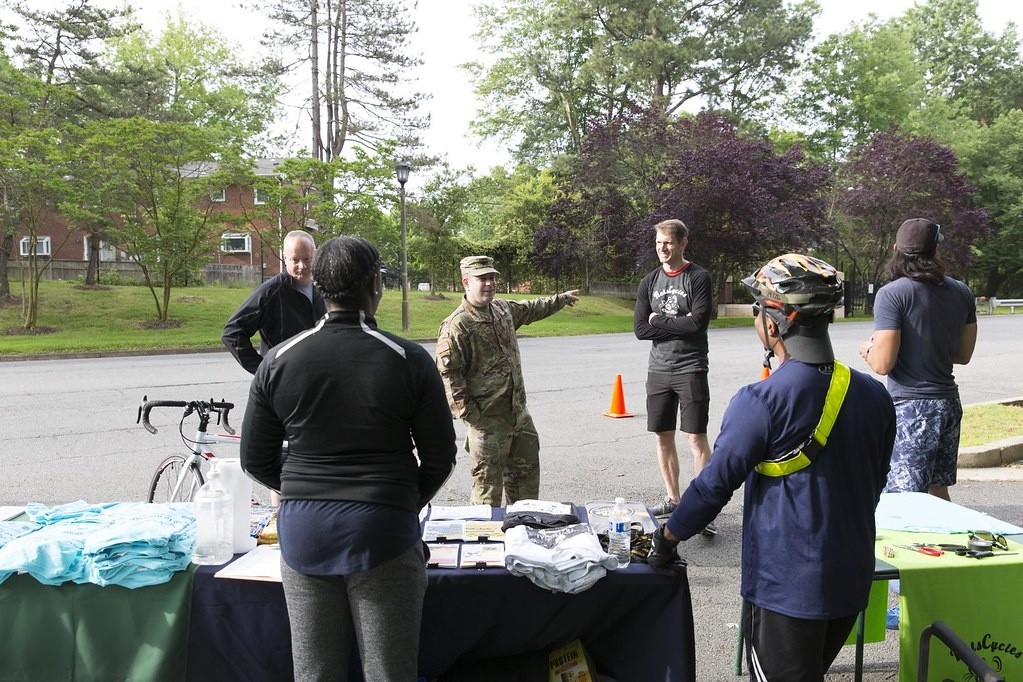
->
[968,530,1008,551]
[751,301,780,325]
[934,224,941,242]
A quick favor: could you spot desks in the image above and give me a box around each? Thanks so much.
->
[0,502,696,682]
[734,491,1023,682]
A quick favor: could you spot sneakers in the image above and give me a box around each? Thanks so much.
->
[649,496,680,518]
[704,521,718,536]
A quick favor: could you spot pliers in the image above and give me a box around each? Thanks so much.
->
[905,545,944,557]
[927,544,966,551]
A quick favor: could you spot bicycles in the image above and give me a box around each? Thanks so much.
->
[136,399,240,505]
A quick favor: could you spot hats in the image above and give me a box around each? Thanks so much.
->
[757,307,834,363]
[460,256,500,276]
[896,218,945,252]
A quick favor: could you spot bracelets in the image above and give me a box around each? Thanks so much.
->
[865,346,873,361]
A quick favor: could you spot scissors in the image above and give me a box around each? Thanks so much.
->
[955,548,1019,560]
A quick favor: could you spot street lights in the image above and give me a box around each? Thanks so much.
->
[397,162,411,331]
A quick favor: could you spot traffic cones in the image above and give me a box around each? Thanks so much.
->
[604,373,631,418]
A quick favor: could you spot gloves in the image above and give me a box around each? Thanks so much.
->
[646,523,688,573]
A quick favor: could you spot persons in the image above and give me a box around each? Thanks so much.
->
[860,218,976,503]
[240,237,458,682]
[985,283,997,315]
[631,253,896,682]
[633,219,718,535]
[221,230,328,506]
[435,256,579,507]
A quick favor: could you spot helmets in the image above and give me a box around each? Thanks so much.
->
[741,253,844,319]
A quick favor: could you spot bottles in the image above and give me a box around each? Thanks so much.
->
[606,497,632,568]
[192,458,233,564]
